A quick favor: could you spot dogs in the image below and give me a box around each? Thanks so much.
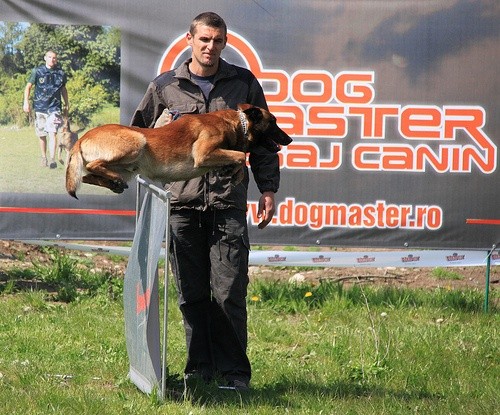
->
[53,109,87,170]
[65,102,292,202]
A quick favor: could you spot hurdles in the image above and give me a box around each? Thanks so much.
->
[123,173,172,401]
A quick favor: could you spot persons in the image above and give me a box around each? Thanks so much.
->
[128,11,281,392]
[23,50,69,167]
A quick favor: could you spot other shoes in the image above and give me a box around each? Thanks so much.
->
[40,158,47,166]
[50,161,57,168]
[166,374,243,395]
